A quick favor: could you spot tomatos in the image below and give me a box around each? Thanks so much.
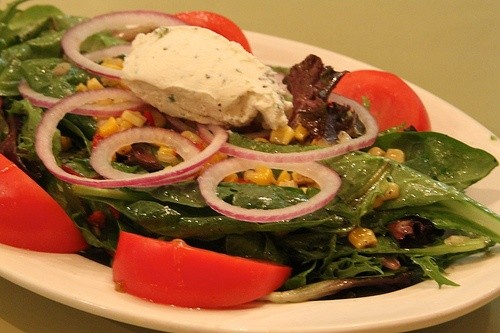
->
[332,69,430,132]
[112,231,295,308]
[0,154,88,253]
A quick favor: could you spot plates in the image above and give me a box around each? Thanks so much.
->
[1,29,500,333]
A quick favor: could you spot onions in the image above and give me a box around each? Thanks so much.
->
[16,9,379,223]
